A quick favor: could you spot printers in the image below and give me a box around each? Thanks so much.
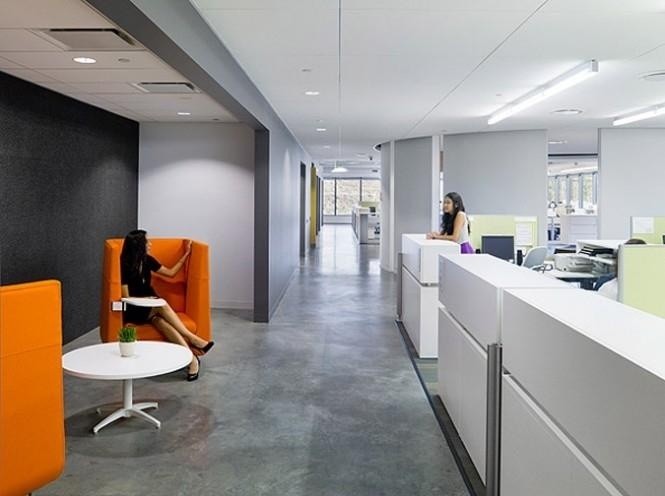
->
[555,252,592,273]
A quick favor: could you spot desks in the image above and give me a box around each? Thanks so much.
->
[515,251,617,290]
[62,340,194,434]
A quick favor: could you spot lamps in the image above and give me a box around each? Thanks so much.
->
[331,166,348,174]
[611,105,665,128]
[487,60,598,126]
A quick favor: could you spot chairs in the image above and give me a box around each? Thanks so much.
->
[520,247,547,271]
[100,238,212,356]
[1,279,66,496]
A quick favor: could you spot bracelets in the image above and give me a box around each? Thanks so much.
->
[178,261,184,264]
[446,236,448,239]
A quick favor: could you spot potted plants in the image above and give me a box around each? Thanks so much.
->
[118,326,137,357]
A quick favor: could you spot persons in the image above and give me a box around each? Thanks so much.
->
[120,230,214,382]
[596,238,646,302]
[547,202,559,241]
[427,192,474,254]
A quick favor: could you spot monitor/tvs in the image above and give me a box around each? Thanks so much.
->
[481,235,515,261]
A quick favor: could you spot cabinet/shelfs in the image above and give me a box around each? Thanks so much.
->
[559,215,597,241]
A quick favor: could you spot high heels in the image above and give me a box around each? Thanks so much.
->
[191,342,214,354]
[188,355,200,382]
